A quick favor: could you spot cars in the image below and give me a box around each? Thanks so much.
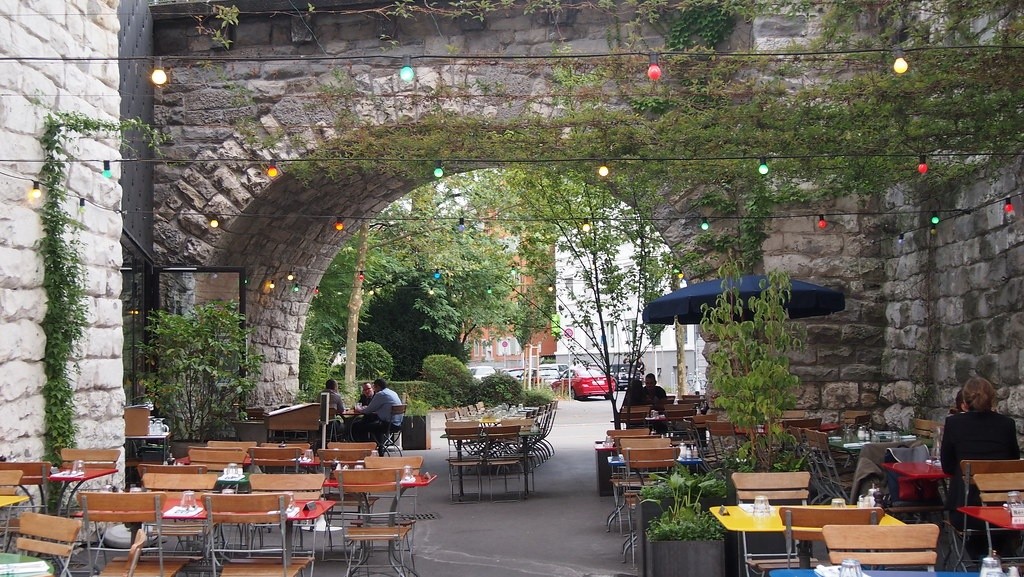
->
[603,364,644,390]
[551,365,616,401]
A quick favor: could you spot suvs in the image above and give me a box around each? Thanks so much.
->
[501,363,568,388]
[468,366,497,384]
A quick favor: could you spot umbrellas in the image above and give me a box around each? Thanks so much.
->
[642,274,845,374]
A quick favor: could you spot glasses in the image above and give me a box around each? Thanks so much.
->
[373,386,376,390]
[363,388,371,392]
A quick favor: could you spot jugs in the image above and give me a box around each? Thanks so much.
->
[841,424,856,443]
[152,417,169,435]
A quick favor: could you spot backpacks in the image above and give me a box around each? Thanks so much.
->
[884,445,940,501]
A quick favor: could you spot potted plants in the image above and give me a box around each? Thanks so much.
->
[123,233,811,577]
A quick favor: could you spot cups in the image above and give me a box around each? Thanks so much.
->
[403,465,413,481]
[839,559,864,577]
[71,459,86,477]
[831,498,846,509]
[475,402,523,421]
[227,462,238,478]
[1007,492,1021,509]
[305,450,315,462]
[286,493,296,512]
[606,436,613,447]
[371,450,379,457]
[180,491,196,511]
[355,403,362,410]
[753,495,771,517]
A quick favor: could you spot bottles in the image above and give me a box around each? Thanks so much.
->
[686,447,691,458]
[864,488,880,507]
[857,426,865,440]
[651,410,659,418]
[857,498,863,508]
[693,446,698,458]
[979,555,1019,577]
[865,428,898,443]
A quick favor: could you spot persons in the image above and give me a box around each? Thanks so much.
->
[695,391,704,404]
[940,375,1020,563]
[623,372,668,425]
[317,378,403,441]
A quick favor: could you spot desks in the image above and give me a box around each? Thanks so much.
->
[770,568,1021,577]
[607,455,703,534]
[124,431,173,466]
[18,469,119,554]
[175,455,320,474]
[322,474,437,560]
[880,462,956,568]
[954,506,1024,572]
[710,504,908,577]
[593,441,622,507]
[0,494,30,552]
[737,421,845,469]
[818,434,933,503]
[337,409,377,443]
[72,500,335,577]
[199,471,265,559]
[0,553,54,577]
[440,406,542,500]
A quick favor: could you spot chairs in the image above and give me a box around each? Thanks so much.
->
[0,392,1024,577]
